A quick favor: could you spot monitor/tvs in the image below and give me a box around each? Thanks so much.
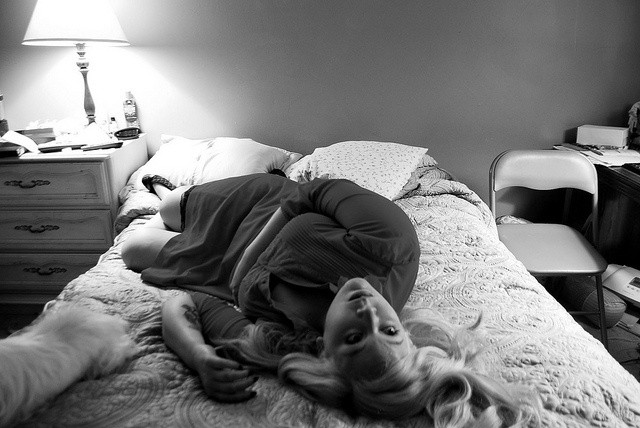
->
[0,143,28,157]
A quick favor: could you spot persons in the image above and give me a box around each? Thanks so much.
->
[119,172,543,427]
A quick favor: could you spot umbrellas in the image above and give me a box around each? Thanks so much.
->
[142,174,177,197]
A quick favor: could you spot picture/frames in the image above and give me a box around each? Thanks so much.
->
[554,142,639,228]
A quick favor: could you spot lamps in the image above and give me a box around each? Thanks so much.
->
[488,149,609,353]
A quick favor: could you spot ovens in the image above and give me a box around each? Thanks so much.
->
[0,136,640,428]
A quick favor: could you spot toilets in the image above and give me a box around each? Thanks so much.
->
[123,93,142,134]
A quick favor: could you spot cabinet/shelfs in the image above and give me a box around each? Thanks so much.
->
[123,90,141,133]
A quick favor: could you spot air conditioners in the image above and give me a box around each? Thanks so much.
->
[1,298,146,427]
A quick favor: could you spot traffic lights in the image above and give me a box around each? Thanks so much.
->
[284,140,428,202]
[118,136,303,205]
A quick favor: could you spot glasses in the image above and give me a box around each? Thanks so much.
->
[20,0,132,147]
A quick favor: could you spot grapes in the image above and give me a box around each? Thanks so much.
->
[0,133,149,305]
